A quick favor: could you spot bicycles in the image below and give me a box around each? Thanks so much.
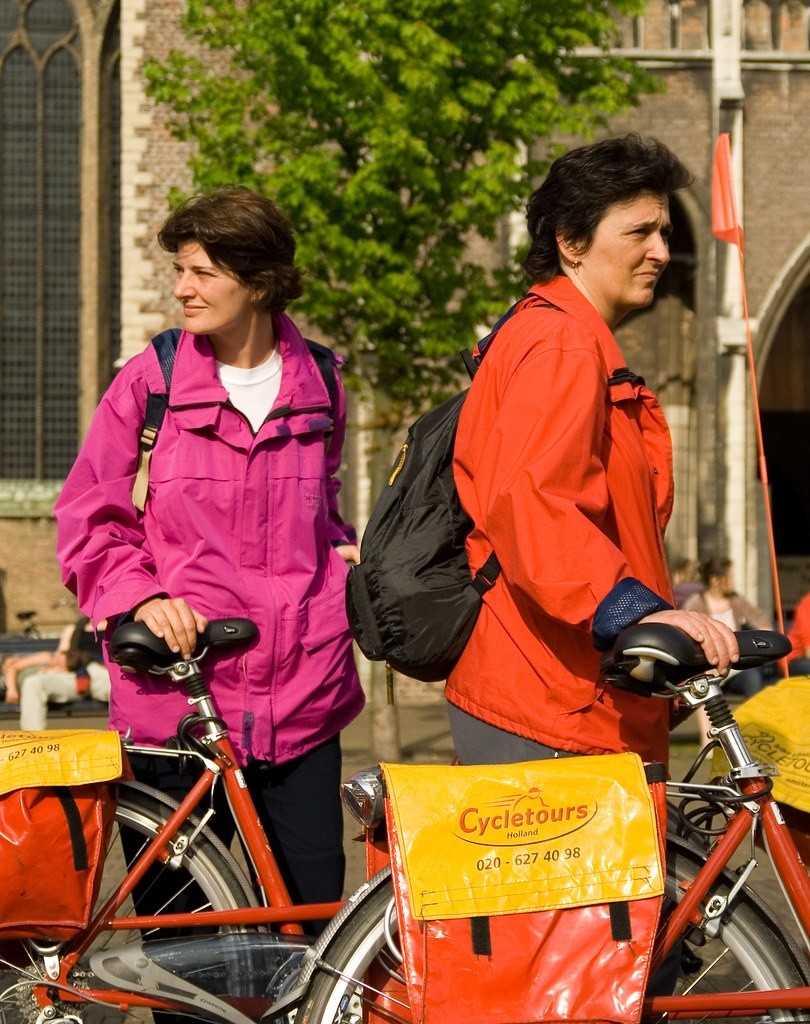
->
[1,616,712,1024]
[257,622,810,1024]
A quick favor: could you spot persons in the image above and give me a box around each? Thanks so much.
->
[442,131,742,1024]
[53,182,361,1024]
[0,618,110,731]
[679,555,810,698]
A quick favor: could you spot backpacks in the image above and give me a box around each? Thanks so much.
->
[345,298,564,683]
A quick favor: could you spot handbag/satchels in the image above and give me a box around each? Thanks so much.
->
[356,750,665,1024]
[0,729,124,971]
